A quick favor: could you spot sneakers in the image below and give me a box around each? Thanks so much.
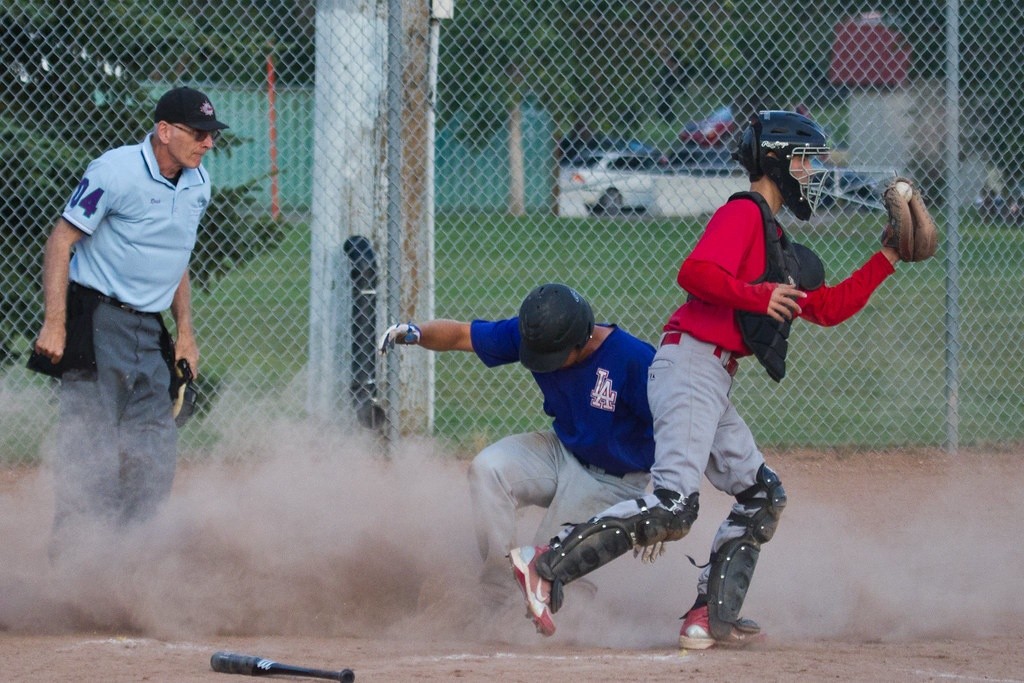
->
[678,606,768,649]
[505,546,556,636]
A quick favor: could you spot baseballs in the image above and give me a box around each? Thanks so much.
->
[895,180,913,203]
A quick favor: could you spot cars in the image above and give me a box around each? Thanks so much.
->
[976,187,1023,223]
[555,104,887,215]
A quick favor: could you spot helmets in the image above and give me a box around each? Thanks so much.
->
[732,110,826,175]
[518,283,594,374]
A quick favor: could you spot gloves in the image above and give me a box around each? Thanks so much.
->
[378,323,421,358]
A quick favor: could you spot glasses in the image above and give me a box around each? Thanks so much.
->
[170,124,221,141]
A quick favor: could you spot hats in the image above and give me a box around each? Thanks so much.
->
[154,85,230,131]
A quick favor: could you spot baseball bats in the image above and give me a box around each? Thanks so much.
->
[207,651,355,683]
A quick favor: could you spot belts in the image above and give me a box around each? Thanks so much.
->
[661,333,740,377]
[574,454,625,480]
[98,295,143,316]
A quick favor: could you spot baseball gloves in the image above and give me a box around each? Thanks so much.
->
[885,175,937,264]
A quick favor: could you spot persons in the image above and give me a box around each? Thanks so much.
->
[377,281,666,652]
[503,107,916,652]
[34,85,230,622]
